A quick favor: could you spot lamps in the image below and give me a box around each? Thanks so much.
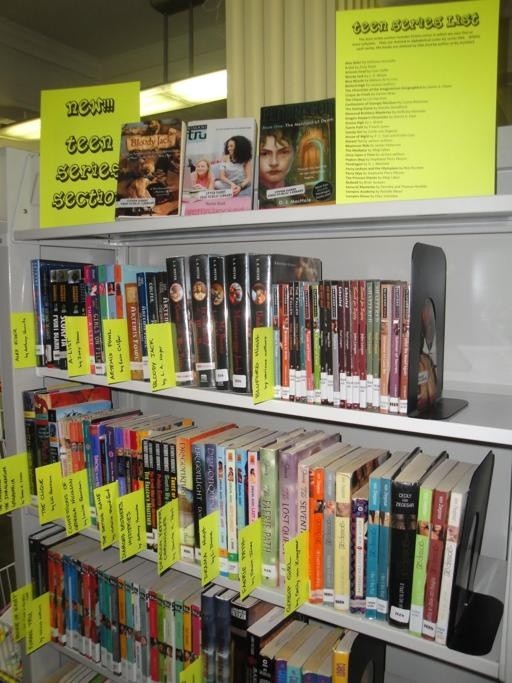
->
[3,62,229,139]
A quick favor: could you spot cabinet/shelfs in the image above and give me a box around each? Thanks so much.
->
[4,137,511,681]
